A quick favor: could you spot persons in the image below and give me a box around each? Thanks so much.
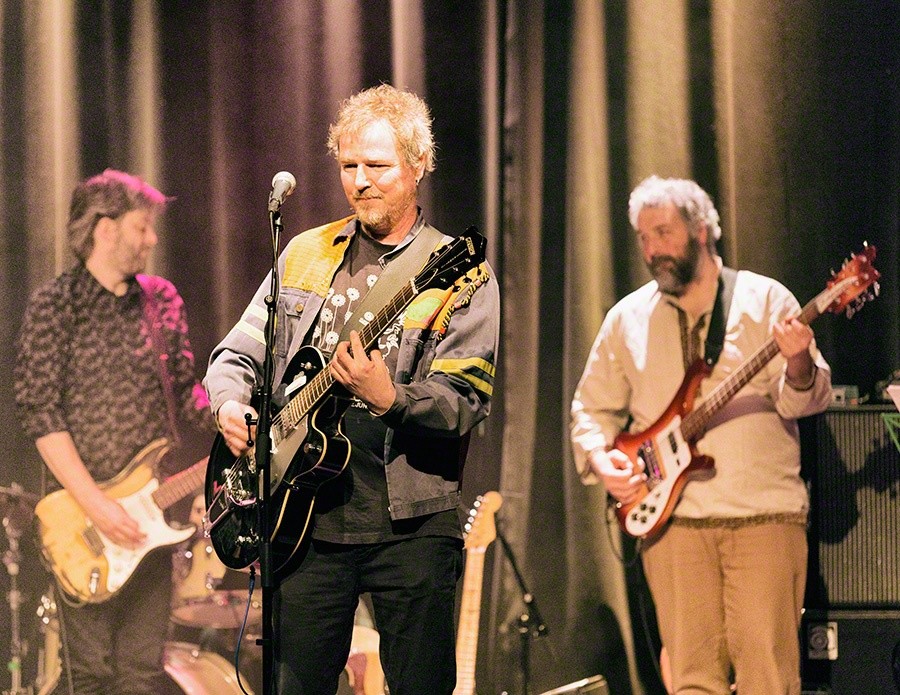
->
[14,170,218,694]
[204,84,499,695]
[569,175,831,694]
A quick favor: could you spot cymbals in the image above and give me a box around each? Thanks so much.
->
[0,486,38,503]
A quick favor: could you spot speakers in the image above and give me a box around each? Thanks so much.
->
[794,405,899,607]
[798,608,900,695]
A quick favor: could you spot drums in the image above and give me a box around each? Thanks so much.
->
[169,493,265,631]
[37,640,257,695]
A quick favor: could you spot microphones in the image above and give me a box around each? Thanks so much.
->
[268,171,297,214]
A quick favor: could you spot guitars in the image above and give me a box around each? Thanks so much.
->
[203,222,489,581]
[34,437,210,606]
[605,239,882,544]
[451,486,505,695]
